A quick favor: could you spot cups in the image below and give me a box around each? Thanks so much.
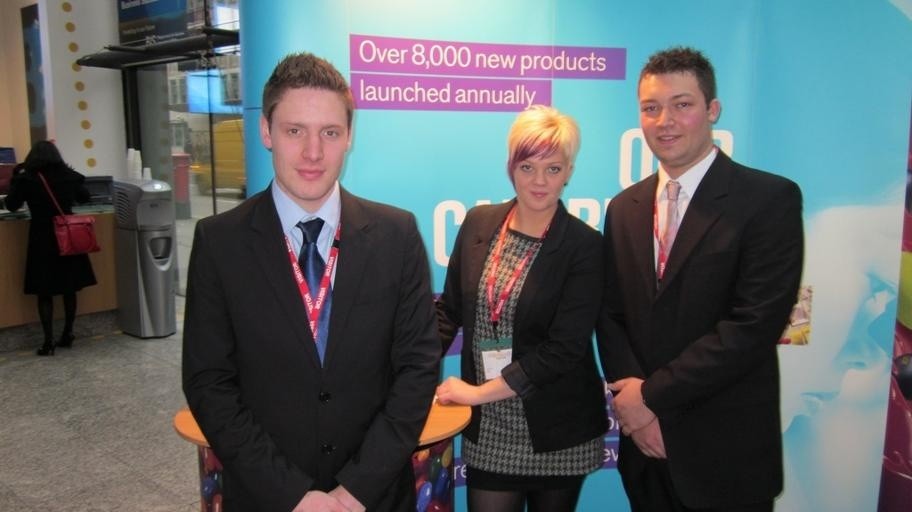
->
[127,148,142,179]
[144,167,152,181]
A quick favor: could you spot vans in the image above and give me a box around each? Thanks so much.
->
[190,119,246,197]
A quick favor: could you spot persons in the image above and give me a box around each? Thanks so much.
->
[590,45,806,509]
[5,139,93,356]
[173,48,446,512]
[441,99,610,510]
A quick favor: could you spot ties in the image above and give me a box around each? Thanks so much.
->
[294,216,334,369]
[656,179,682,293]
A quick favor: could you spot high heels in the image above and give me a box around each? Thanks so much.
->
[37,345,56,357]
[56,332,75,350]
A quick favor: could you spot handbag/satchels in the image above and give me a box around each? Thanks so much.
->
[53,214,103,257]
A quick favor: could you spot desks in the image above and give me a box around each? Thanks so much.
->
[174,405,473,511]
